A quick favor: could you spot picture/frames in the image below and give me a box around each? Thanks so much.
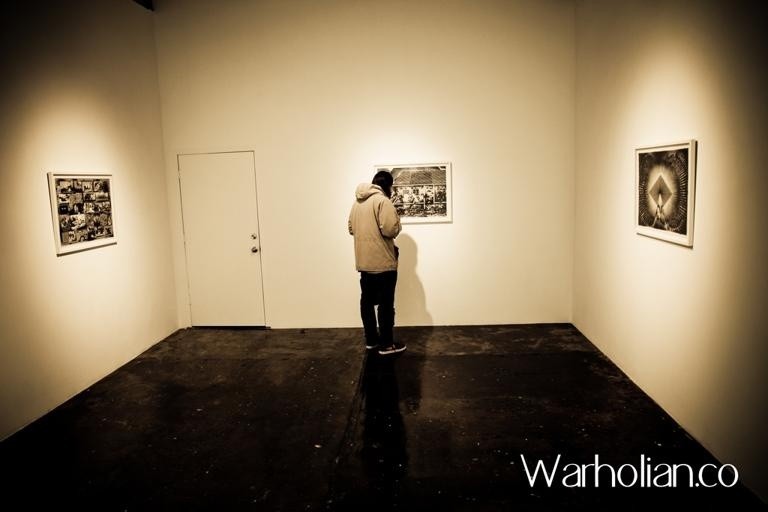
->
[46,170,119,258]
[371,159,454,227]
[633,138,698,252]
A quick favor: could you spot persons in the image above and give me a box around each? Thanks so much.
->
[347,170,406,355]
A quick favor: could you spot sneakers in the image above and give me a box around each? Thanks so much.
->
[365,337,379,350]
[378,342,407,355]
